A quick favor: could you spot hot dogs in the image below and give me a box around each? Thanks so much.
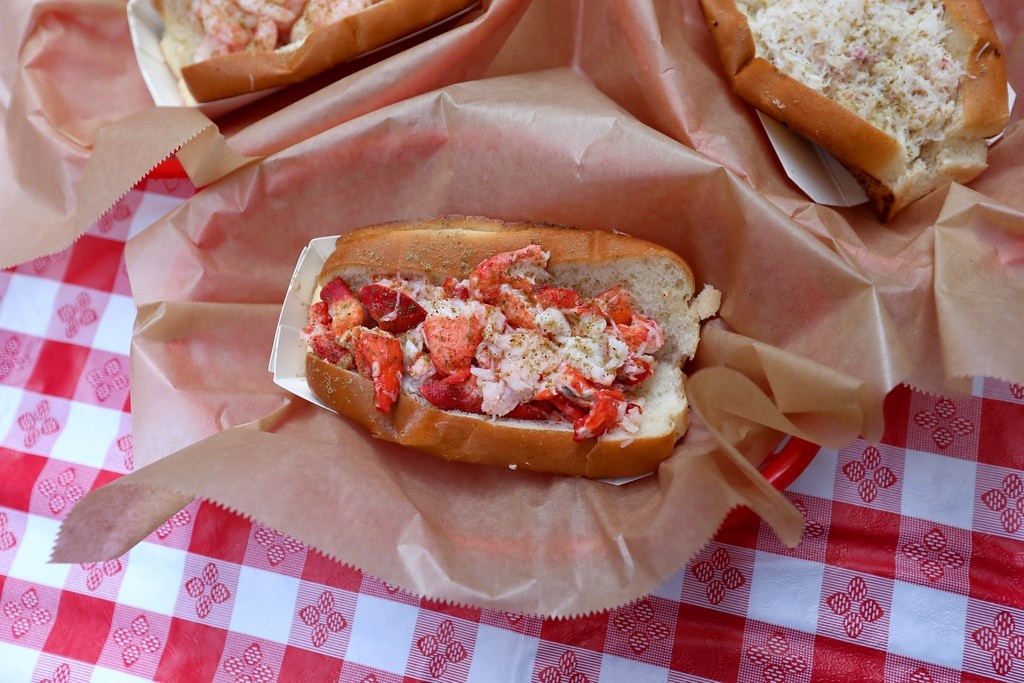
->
[152,0,478,104]
[299,215,724,480]
[699,0,1009,221]
